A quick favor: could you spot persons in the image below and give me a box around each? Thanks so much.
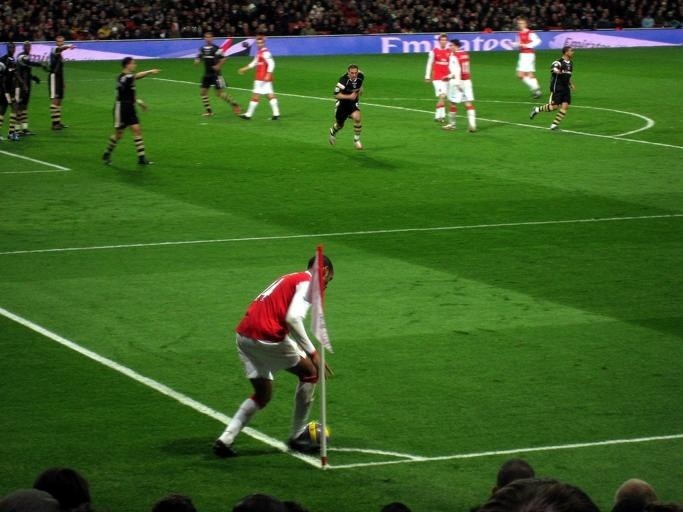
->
[235,34,282,120]
[453,0,507,32]
[442,39,477,132]
[613,478,682,511]
[212,255,333,459]
[477,459,601,511]
[503,18,543,100]
[538,0,683,30]
[506,0,543,31]
[233,492,302,511]
[382,502,410,511]
[0,42,22,141]
[1,488,61,511]
[531,46,575,130]
[47,37,77,129]
[425,0,453,32]
[329,65,364,150]
[283,0,424,37]
[32,467,94,510]
[0,0,193,41]
[104,57,163,166]
[424,35,454,123]
[15,41,50,137]
[153,495,196,511]
[193,33,242,117]
[194,0,283,38]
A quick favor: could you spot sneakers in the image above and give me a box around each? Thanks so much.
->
[552,125,560,130]
[270,113,280,119]
[238,113,251,118]
[435,114,445,120]
[52,124,66,129]
[442,123,456,129]
[355,140,363,151]
[330,134,335,146]
[529,106,537,119]
[103,156,113,165]
[468,127,477,130]
[217,433,238,456]
[529,89,542,97]
[138,159,154,165]
[290,437,324,453]
[22,128,35,134]
[202,111,214,117]
[234,106,240,115]
[8,132,21,139]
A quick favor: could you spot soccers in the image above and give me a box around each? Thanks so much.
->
[303,420,332,445]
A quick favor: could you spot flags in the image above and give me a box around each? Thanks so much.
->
[305,246,336,354]
